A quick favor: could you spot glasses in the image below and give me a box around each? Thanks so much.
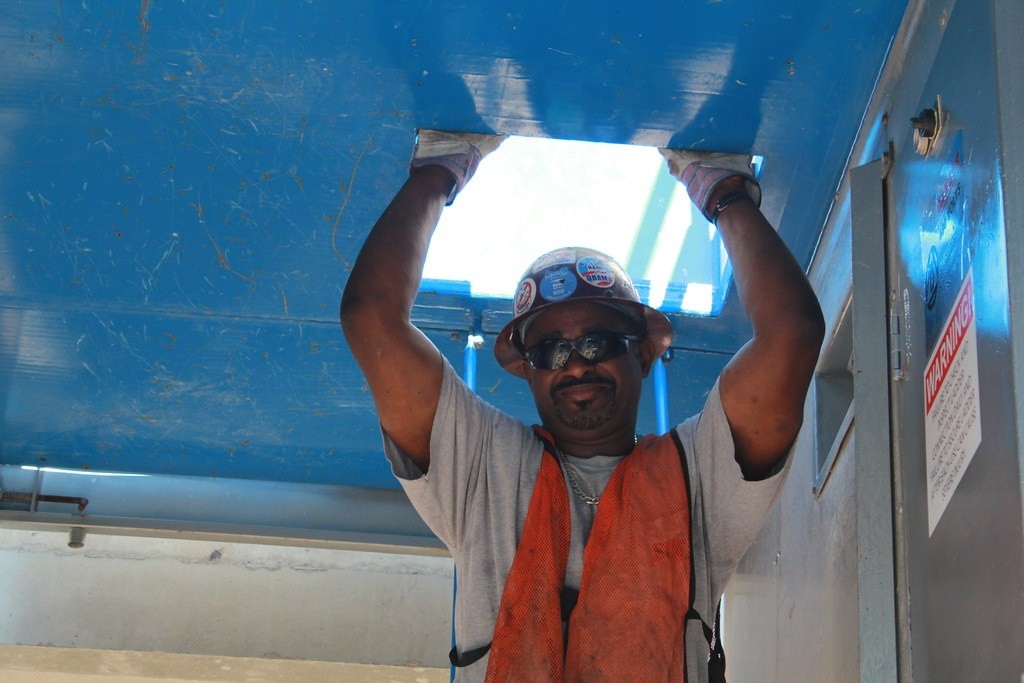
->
[523,330,645,373]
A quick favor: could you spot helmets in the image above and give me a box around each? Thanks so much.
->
[493,247,675,380]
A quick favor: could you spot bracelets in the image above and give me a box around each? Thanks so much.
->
[711,189,757,226]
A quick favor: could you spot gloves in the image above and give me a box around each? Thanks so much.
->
[410,128,510,207]
[659,147,762,225]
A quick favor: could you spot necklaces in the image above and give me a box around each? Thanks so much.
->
[553,433,645,508]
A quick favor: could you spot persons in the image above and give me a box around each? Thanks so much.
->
[338,128,827,683]
